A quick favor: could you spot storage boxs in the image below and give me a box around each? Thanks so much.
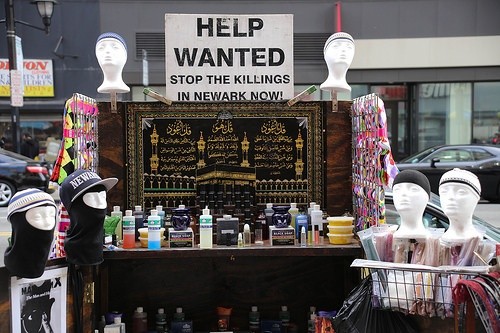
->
[168,228,195,248]
[269,225,296,245]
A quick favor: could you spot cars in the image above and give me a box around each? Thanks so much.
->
[0,147,50,207]
[383,187,500,257]
[395,144,500,204]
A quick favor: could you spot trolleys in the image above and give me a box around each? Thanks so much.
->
[350,258,500,333]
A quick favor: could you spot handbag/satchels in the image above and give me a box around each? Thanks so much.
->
[332,273,419,333]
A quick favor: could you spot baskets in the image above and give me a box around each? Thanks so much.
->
[363,266,481,321]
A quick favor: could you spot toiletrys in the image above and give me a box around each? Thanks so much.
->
[107,204,329,249]
[98,303,336,332]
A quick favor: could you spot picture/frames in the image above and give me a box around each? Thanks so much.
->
[125,101,327,224]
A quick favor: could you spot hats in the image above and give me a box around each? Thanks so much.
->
[439,167,482,199]
[7,188,56,220]
[95,32,128,50]
[392,169,430,199]
[59,168,119,211]
[325,32,354,51]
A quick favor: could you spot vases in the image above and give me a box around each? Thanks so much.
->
[170,209,192,230]
[271,205,292,228]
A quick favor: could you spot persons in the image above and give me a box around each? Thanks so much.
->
[439,168,482,244]
[56,170,120,264]
[96,33,131,95]
[319,31,354,92]
[6,187,56,281]
[390,170,431,236]
[21,131,39,159]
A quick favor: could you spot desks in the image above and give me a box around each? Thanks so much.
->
[101,237,364,321]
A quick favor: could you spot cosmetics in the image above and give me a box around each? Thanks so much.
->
[94,306,318,333]
